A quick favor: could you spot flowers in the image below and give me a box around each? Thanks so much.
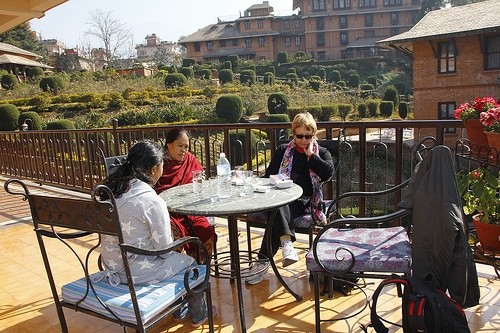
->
[456,166,500,223]
[453,97,500,133]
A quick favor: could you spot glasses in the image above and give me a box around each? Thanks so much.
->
[295,132,314,140]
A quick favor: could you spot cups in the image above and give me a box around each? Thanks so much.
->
[193,170,202,194]
[209,177,219,203]
[234,165,244,185]
[243,171,253,200]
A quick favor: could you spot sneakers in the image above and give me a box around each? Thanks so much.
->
[190,305,216,328]
[246,263,271,283]
[282,246,299,267]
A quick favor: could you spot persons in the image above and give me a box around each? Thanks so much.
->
[155,127,216,273]
[96,140,217,327]
[245,112,335,284]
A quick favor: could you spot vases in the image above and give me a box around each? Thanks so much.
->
[463,120,500,164]
[472,214,500,251]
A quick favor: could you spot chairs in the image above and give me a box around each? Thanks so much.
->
[4,178,214,333]
[306,136,452,333]
[246,129,346,269]
[95,139,219,277]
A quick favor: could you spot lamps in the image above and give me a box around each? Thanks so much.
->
[21,119,34,132]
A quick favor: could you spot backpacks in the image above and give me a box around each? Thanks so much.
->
[370,277,471,333]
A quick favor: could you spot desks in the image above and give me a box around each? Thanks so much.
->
[158,176,302,333]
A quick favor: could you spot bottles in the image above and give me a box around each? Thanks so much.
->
[217,153,232,199]
